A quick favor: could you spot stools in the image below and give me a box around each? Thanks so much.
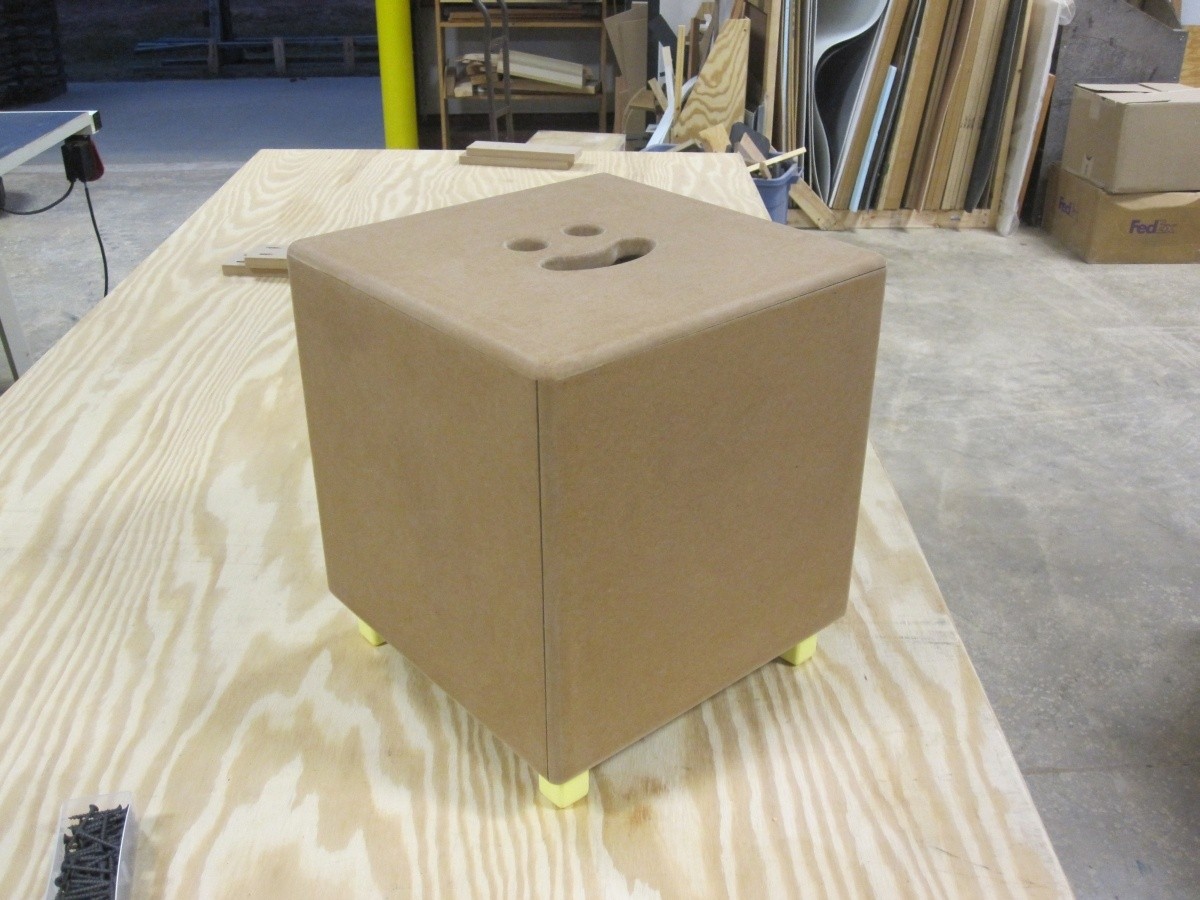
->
[285,173,888,810]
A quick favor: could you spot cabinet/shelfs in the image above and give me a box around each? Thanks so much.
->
[434,0,609,148]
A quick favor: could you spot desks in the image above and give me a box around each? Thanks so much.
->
[0,110,102,380]
[0,148,1074,900]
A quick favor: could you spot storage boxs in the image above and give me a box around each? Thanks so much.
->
[45,790,136,900]
[640,143,804,224]
[1043,79,1200,265]
